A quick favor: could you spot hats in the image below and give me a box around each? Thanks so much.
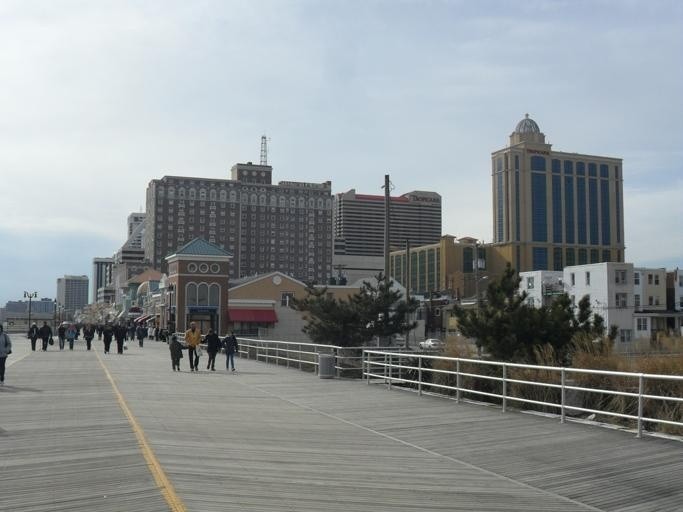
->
[191,322,197,327]
[172,336,177,340]
[32,323,36,326]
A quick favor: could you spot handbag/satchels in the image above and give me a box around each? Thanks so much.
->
[49,338,54,345]
[196,344,203,356]
[8,350,11,354]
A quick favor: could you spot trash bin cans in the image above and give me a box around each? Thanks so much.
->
[319,352,335,379]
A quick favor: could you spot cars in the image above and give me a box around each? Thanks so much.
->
[419,339,447,351]
[395,334,404,345]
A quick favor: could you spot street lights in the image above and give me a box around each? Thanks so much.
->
[23,290,37,329]
[166,282,173,333]
[471,243,488,357]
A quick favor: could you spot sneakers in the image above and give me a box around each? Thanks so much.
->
[178,368,180,370]
[207,366,210,369]
[232,368,235,371]
[211,369,217,371]
[191,368,198,371]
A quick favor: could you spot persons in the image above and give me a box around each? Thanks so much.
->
[26,320,239,373]
[0,323,12,385]
